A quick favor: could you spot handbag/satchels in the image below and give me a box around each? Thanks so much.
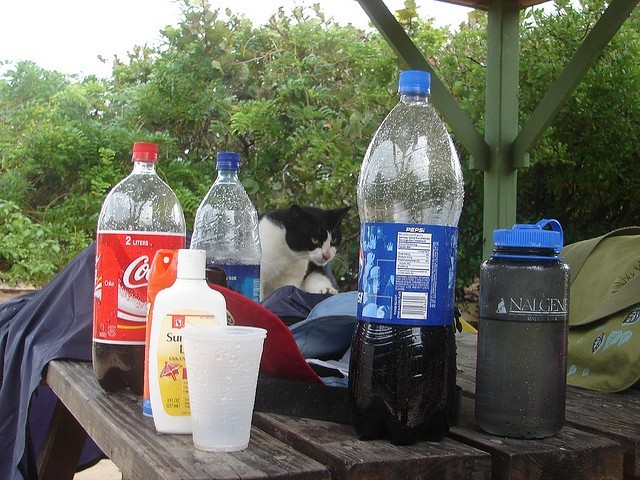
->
[560,226,640,393]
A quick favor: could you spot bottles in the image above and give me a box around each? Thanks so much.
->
[149,249,229,437]
[347,69,466,446]
[92,141,187,397]
[189,151,262,306]
[474,216,568,440]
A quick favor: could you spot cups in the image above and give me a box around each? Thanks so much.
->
[179,325,267,453]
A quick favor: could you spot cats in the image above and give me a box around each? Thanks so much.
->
[257,203,352,306]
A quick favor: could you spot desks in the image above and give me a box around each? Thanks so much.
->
[0,294,640,480]
